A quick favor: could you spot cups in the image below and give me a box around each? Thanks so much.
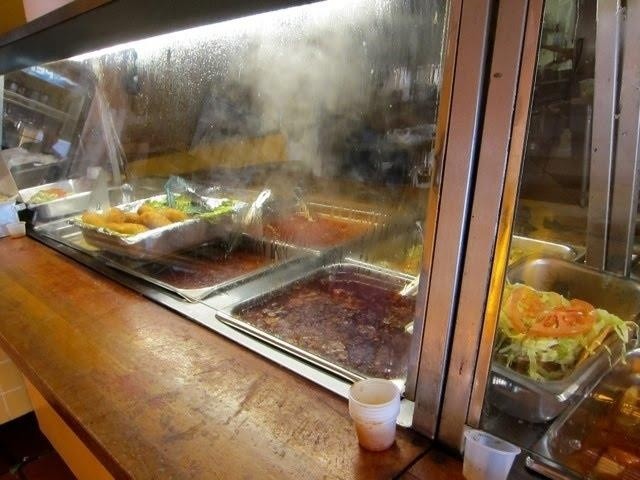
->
[347,377,402,453]
[461,429,524,480]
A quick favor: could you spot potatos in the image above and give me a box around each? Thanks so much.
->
[82,205,188,234]
